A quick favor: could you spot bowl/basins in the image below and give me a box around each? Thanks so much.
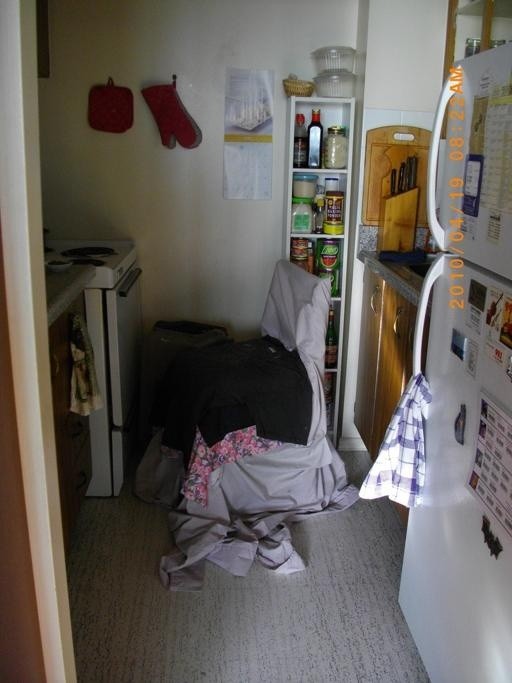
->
[46,260,73,272]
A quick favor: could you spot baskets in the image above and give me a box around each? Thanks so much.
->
[283,79,313,96]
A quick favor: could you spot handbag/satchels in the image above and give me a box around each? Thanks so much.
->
[89,78,133,133]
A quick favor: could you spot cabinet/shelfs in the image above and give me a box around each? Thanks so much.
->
[282,97,356,448]
[355,266,430,465]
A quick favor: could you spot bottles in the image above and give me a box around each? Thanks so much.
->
[291,197,313,234]
[294,113,309,168]
[325,309,338,369]
[324,125,348,169]
[306,108,323,169]
[315,199,326,234]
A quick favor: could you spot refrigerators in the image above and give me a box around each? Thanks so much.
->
[396,41,511,683]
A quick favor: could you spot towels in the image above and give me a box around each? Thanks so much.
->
[358,374,433,509]
[69,314,103,416]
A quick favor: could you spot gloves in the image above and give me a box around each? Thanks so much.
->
[141,85,202,148]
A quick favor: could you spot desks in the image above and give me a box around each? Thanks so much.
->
[48,259,104,329]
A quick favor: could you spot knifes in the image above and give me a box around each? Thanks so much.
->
[391,155,418,198]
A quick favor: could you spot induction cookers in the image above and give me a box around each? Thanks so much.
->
[43,244,135,270]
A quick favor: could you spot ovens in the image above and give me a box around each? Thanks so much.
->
[104,260,143,495]
